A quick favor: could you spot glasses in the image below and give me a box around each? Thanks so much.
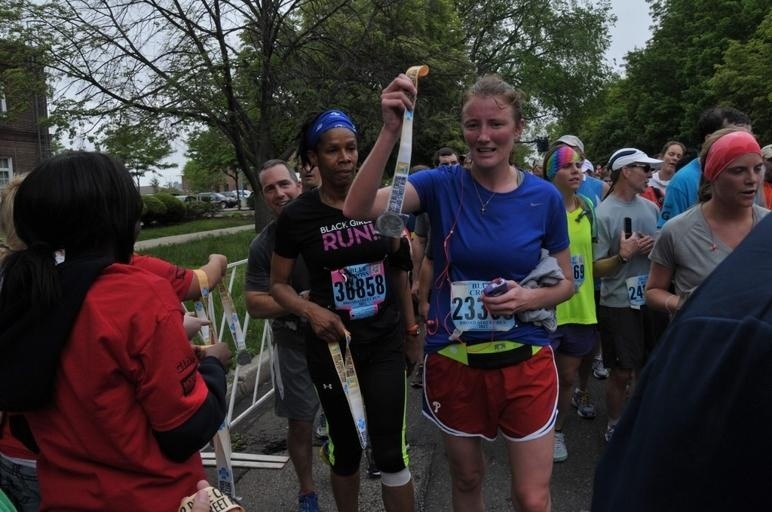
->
[630,164,656,172]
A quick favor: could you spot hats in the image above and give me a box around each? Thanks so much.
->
[606,148,665,176]
[582,159,594,173]
[762,145,772,158]
[551,135,585,154]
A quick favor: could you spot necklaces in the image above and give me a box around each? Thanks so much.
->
[473,170,505,213]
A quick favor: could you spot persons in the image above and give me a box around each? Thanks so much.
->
[533,108,772,511]
[343,79,573,511]
[0,151,242,511]
[245,111,475,511]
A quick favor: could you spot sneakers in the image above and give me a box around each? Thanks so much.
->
[314,411,328,440]
[366,465,381,478]
[570,387,597,418]
[599,421,616,449]
[591,358,609,380]
[552,432,569,462]
[297,491,319,512]
[410,362,423,388]
